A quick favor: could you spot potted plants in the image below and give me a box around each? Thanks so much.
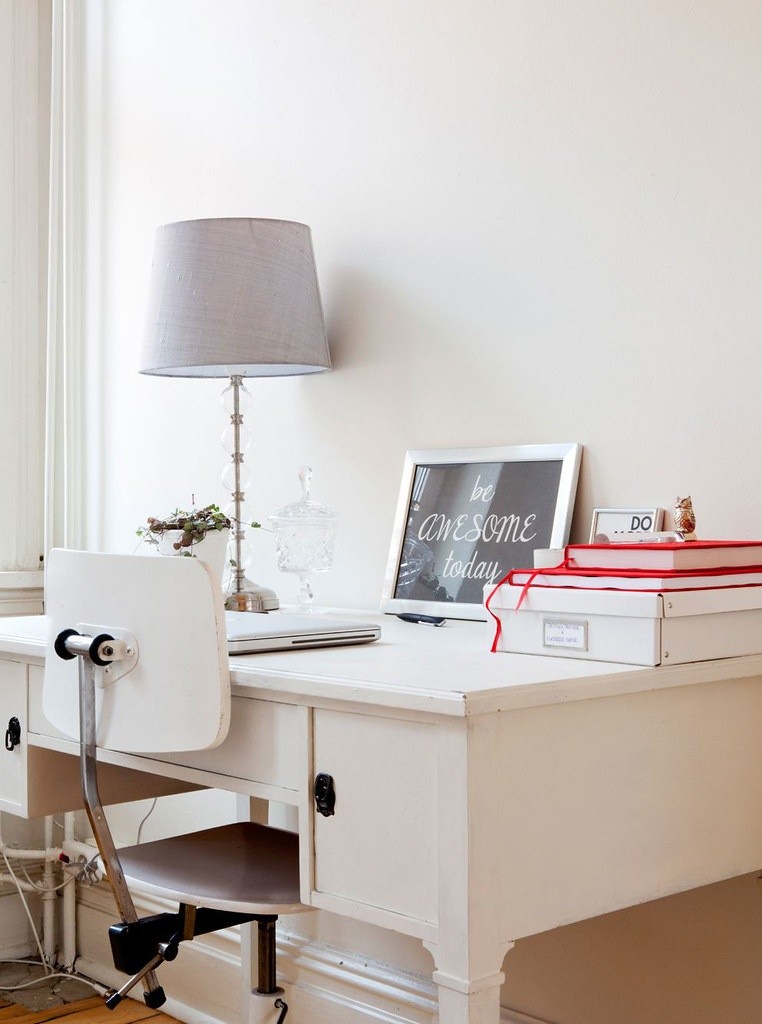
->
[143,498,268,589]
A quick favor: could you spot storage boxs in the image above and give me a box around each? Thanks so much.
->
[483,583,762,666]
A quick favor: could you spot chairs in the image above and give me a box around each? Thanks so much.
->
[40,546,322,1024]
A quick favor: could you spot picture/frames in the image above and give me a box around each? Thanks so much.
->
[589,507,665,545]
[380,443,584,621]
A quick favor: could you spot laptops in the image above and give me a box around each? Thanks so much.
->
[225,611,381,656]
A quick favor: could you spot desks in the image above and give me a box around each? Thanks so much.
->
[0,602,762,1024]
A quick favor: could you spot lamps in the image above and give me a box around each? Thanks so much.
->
[138,218,332,612]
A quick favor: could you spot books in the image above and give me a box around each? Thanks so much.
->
[509,565,762,593]
[564,541,762,573]
